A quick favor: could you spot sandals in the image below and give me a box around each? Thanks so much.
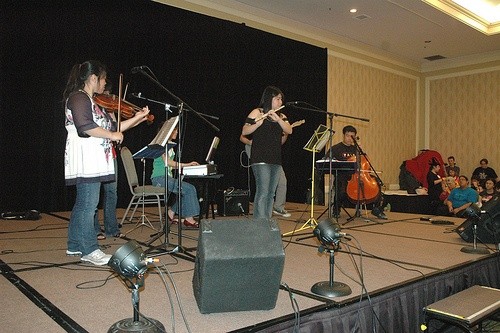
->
[114,233,125,238]
[96,233,106,239]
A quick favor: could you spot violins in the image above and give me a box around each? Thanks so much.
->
[93,93,155,122]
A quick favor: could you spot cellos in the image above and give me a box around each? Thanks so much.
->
[347,136,381,223]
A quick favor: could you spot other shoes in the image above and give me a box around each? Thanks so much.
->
[333,211,340,218]
[372,210,387,219]
[184,219,199,228]
[164,212,177,224]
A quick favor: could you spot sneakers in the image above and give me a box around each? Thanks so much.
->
[273,208,291,217]
[81,249,112,265]
[66,249,82,255]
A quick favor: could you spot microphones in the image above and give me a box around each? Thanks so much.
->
[124,82,129,100]
[285,101,302,107]
[131,66,146,73]
[238,203,244,214]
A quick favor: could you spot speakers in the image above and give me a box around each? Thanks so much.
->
[192,217,285,314]
[455,191,500,244]
[217,189,250,217]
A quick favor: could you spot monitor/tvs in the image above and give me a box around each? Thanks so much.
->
[205,136,221,165]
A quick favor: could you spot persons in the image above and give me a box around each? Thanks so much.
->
[150,124,202,229]
[321,125,389,220]
[62,59,151,267]
[427,156,500,217]
[239,85,292,232]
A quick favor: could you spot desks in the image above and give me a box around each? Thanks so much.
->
[385,194,429,214]
[421,284,500,333]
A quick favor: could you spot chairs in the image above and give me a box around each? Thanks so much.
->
[120,146,166,228]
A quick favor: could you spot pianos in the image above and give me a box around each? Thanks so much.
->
[182,164,218,226]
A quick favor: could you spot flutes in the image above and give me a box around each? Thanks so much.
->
[255,106,285,122]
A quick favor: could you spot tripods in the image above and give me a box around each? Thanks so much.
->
[316,134,384,226]
[129,73,221,261]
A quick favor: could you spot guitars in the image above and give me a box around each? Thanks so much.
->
[245,120,305,158]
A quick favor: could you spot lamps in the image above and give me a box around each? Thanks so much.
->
[106,240,166,333]
[310,217,352,297]
[460,205,491,254]
